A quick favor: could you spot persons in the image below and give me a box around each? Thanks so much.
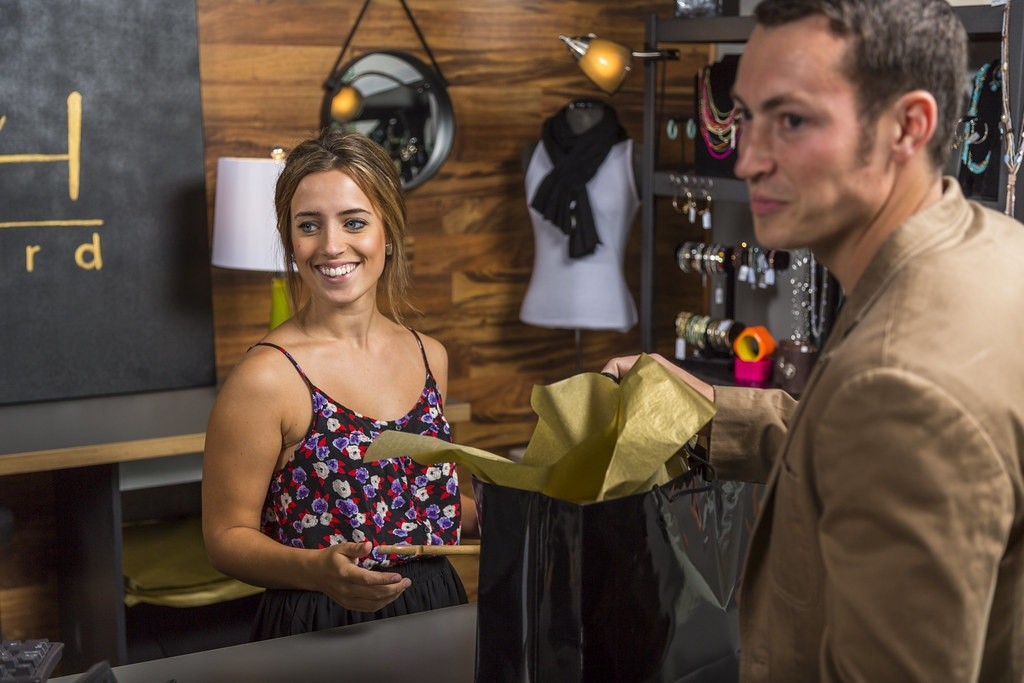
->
[520,97,641,332]
[600,0,1024,683]
[202,132,480,641]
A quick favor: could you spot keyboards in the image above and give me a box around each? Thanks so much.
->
[0,638,64,683]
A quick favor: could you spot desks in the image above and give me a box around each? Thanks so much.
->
[0,365,474,669]
[45,603,478,683]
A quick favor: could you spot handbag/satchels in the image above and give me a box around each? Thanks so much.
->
[474,371,740,683]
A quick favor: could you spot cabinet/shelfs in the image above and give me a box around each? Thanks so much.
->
[639,0,1024,393]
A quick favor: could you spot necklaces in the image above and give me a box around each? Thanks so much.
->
[811,253,827,337]
[698,64,751,159]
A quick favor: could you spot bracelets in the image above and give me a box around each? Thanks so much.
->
[672,241,777,353]
